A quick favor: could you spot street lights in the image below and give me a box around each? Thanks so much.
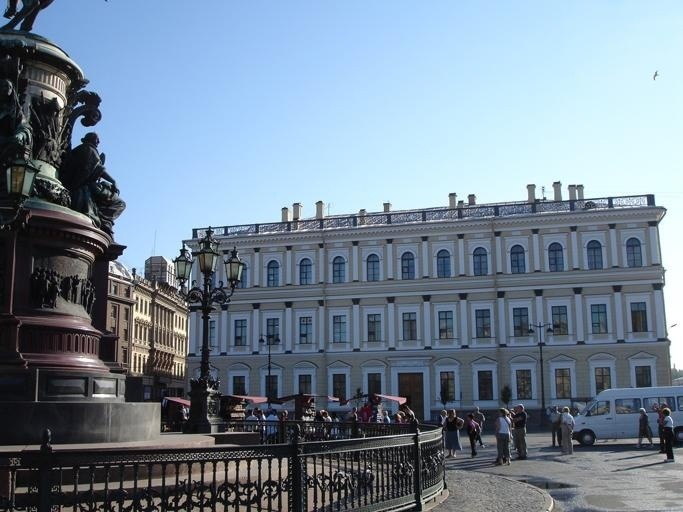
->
[170,225,247,434]
[526,322,554,431]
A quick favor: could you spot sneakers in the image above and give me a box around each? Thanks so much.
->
[635,443,674,463]
[445,454,457,458]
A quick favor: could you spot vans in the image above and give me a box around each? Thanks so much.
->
[571,386,683,447]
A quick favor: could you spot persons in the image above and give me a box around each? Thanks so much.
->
[652,401,669,453]
[31,266,97,314]
[314,401,415,437]
[657,407,675,462]
[438,403,529,466]
[176,404,188,431]
[243,406,289,443]
[549,405,561,448]
[559,406,576,455]
[633,407,655,449]
[0,78,34,164]
[61,131,127,233]
